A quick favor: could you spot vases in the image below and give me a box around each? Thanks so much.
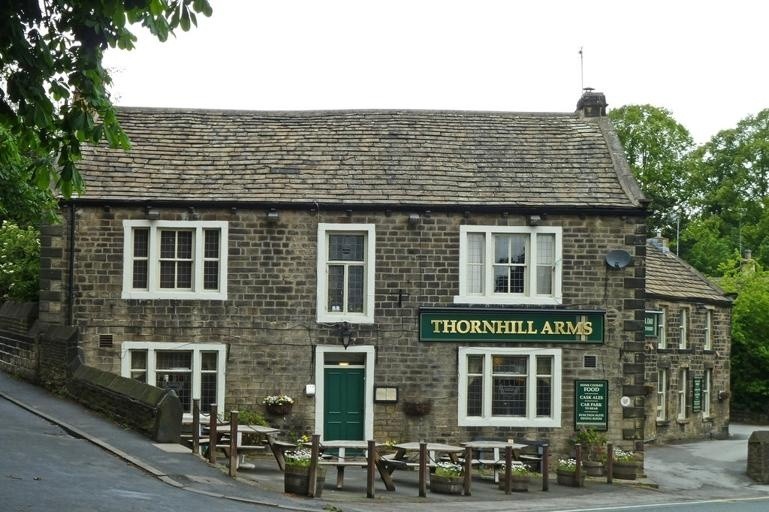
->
[266,404,293,415]
[282,463,327,497]
[498,472,529,492]
[612,463,639,479]
[556,468,585,486]
[429,472,466,494]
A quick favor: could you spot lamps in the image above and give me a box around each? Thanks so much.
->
[338,321,353,350]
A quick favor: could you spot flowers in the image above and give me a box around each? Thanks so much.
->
[556,457,583,471]
[613,448,641,464]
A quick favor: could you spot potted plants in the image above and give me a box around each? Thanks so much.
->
[262,394,295,407]
[565,429,609,476]
[223,409,278,454]
